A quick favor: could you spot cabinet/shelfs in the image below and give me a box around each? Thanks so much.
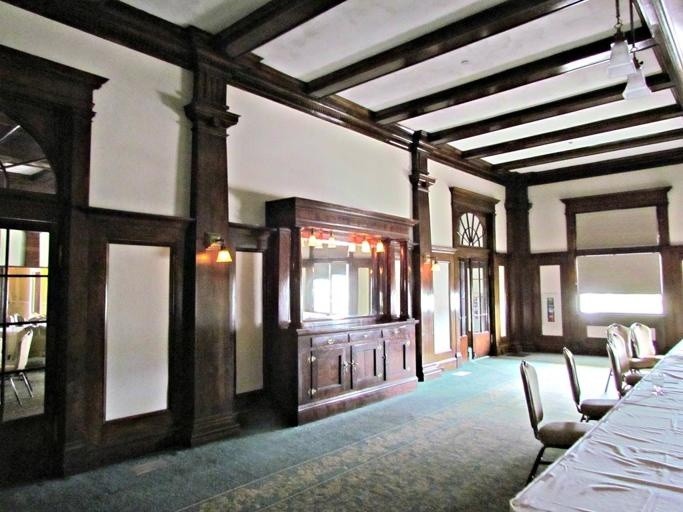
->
[264,197,418,427]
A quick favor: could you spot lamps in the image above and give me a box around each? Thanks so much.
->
[607,1,652,101]
[204,232,232,262]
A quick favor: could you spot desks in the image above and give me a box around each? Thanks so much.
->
[508,338,683,509]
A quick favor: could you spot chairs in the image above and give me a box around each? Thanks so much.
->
[0,326,34,408]
[520,322,665,487]
